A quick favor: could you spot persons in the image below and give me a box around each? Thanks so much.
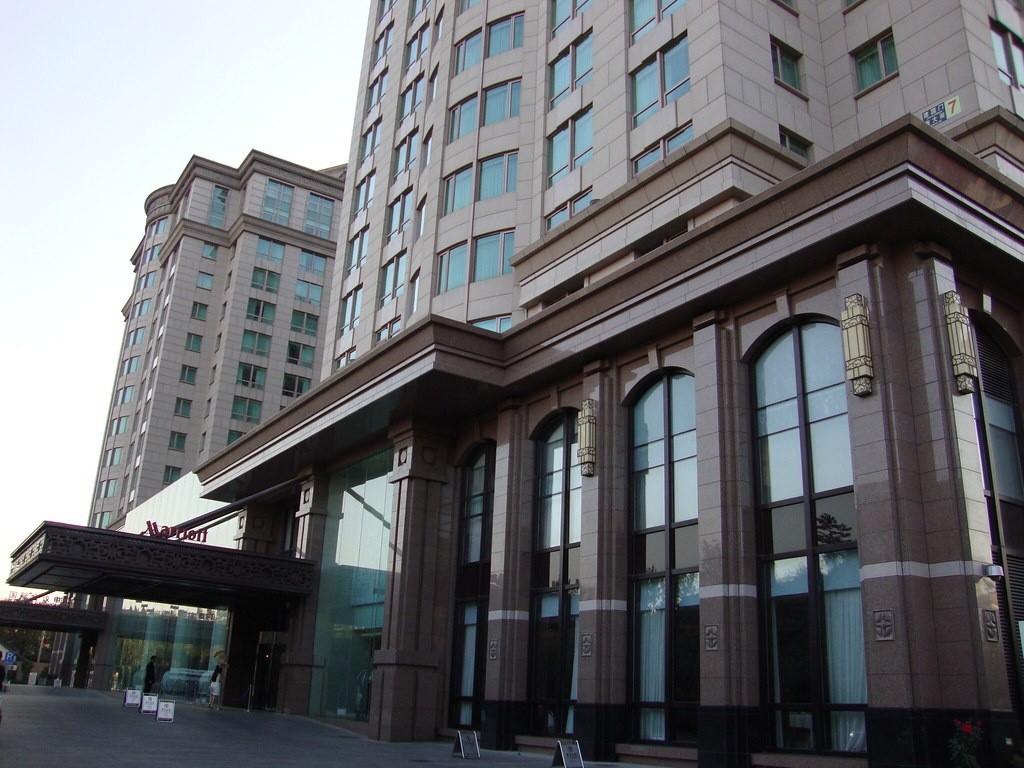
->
[0,652,6,725]
[208,664,223,709]
[145,655,157,696]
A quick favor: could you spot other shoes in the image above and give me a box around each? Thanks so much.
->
[208,703,213,709]
